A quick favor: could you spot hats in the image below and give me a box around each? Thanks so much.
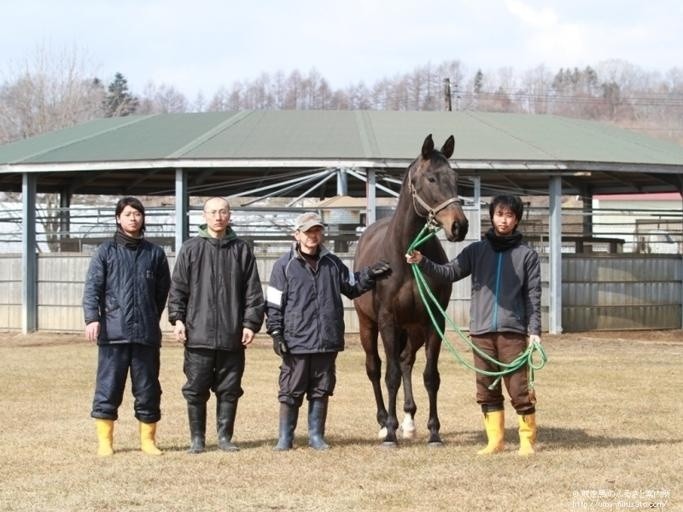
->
[294,212,325,233]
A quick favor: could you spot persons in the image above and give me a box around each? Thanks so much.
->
[81,196,171,458]
[261,210,393,451]
[165,195,267,455]
[403,193,543,457]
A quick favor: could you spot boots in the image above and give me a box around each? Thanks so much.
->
[277,400,298,450]
[307,400,331,450]
[139,422,165,458]
[514,412,535,456]
[187,401,207,454]
[214,400,240,452]
[476,410,505,456]
[94,416,115,458]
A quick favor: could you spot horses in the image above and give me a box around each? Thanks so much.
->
[353,133,469,448]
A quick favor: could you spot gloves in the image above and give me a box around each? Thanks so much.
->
[368,259,390,279]
[272,330,289,357]
